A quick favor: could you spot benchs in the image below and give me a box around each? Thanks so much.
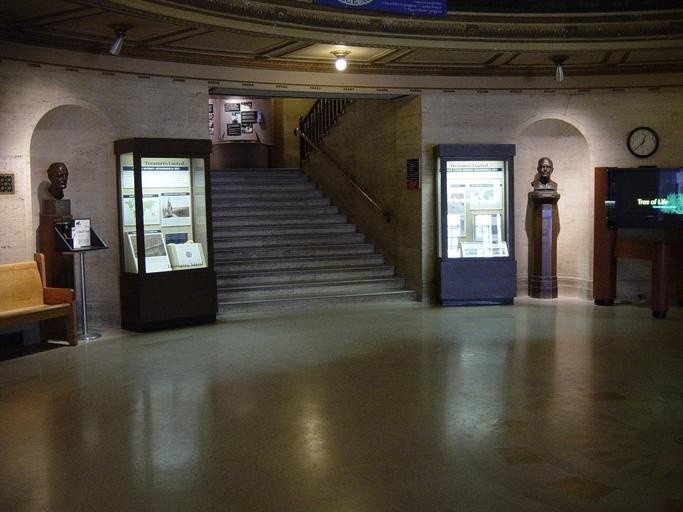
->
[0,253,78,345]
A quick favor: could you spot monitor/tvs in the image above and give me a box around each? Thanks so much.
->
[608,167,683,230]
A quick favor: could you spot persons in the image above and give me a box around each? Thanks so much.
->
[47,162,68,199]
[533,157,558,192]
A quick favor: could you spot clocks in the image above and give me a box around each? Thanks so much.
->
[627,127,659,158]
